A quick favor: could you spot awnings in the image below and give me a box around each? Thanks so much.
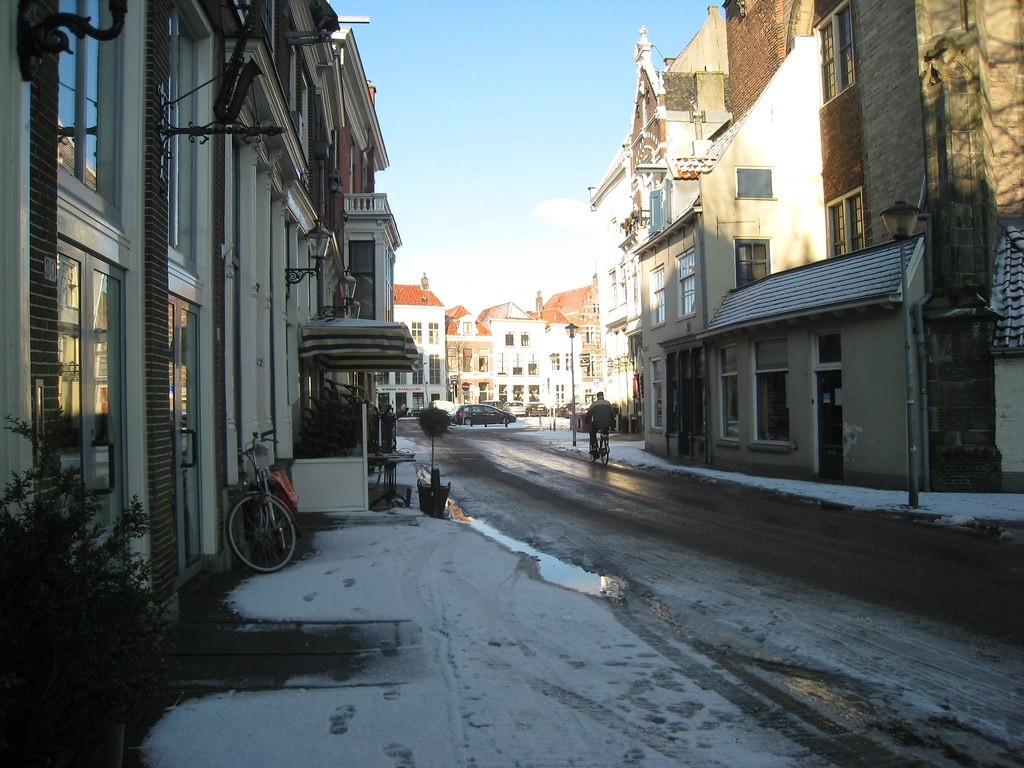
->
[299,317,418,374]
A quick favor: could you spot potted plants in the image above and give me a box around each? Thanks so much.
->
[416,406,451,515]
[625,218,632,225]
[620,223,628,229]
[630,211,639,219]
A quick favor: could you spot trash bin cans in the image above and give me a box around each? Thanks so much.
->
[569,411,593,433]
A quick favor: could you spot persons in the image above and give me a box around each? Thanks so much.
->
[586,392,614,456]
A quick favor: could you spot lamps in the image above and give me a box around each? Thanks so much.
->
[323,299,361,318]
[285,213,333,285]
[309,266,360,319]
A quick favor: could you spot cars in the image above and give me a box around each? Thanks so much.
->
[455,404,516,426]
[480,400,503,409]
[575,402,593,414]
[526,402,548,417]
[503,401,526,417]
[556,402,581,418]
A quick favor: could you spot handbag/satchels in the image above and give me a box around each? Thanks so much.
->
[271,471,299,513]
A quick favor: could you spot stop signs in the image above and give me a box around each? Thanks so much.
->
[462,382,471,392]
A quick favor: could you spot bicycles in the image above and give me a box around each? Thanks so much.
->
[227,428,301,575]
[589,421,610,466]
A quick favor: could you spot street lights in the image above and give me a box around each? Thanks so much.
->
[565,323,579,446]
[878,198,923,510]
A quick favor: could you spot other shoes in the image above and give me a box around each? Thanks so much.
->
[589,449,596,454]
